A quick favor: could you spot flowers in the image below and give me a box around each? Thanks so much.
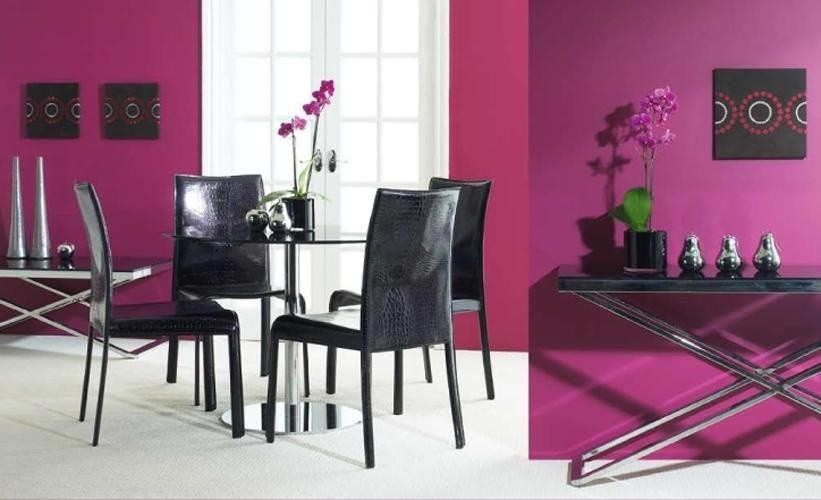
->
[252,79,336,205]
[57,243,75,254]
[607,83,680,232]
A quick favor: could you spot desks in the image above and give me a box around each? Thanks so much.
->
[0,253,174,359]
[557,264,821,486]
[165,223,367,433]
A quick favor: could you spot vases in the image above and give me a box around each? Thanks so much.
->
[59,254,72,259]
[6,155,52,260]
[280,196,316,242]
[621,231,666,272]
[268,202,291,242]
[244,209,267,241]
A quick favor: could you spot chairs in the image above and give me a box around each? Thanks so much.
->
[267,186,464,467]
[168,175,307,385]
[74,181,246,444]
[328,177,494,414]
